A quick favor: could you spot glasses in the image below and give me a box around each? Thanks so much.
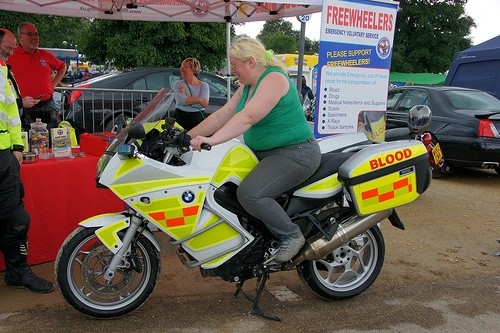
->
[229,61,244,70]
[20,32,40,36]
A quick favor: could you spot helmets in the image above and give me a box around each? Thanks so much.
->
[408,104,433,134]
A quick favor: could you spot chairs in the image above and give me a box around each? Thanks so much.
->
[408,96,427,109]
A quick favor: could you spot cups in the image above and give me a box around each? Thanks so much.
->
[22,153,38,164]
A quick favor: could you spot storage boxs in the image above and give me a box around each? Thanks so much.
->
[79,132,116,157]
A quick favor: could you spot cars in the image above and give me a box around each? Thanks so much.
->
[63,66,241,131]
[383,86,496,176]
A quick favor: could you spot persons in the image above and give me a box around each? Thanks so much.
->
[185,35,322,263]
[51,65,133,87]
[6,22,67,132]
[388,83,394,89]
[172,58,210,133]
[0,27,24,132]
[301,75,314,99]
[405,82,414,86]
[0,60,57,294]
[214,72,239,84]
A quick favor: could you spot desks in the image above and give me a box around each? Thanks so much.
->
[0,150,127,271]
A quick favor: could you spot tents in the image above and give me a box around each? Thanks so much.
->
[0,0,322,103]
[389,72,448,86]
[444,35,500,101]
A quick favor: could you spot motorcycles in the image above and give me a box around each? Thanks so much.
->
[300,88,445,195]
[55,87,444,319]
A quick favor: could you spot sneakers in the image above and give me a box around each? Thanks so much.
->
[274,234,306,261]
[4,265,56,293]
